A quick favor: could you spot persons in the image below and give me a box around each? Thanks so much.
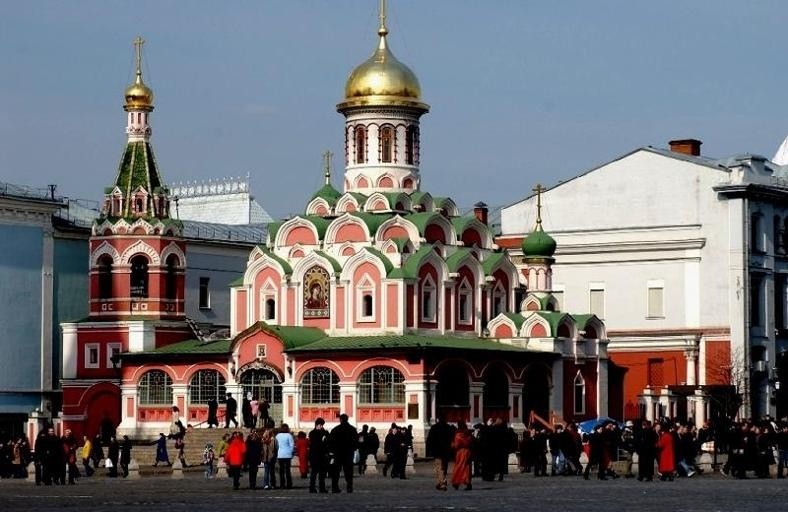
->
[1,393,787,493]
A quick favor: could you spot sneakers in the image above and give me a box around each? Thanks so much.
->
[688,471,696,478]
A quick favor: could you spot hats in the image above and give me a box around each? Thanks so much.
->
[625,420,634,427]
